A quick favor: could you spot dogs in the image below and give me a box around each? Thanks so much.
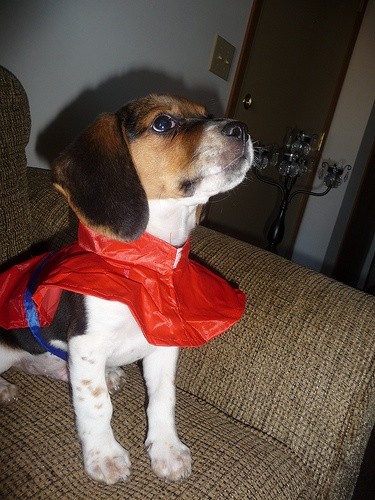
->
[1,92,269,486]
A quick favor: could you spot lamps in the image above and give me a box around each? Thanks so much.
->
[249,127,353,254]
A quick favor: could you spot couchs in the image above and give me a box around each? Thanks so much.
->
[0,65,375,500]
